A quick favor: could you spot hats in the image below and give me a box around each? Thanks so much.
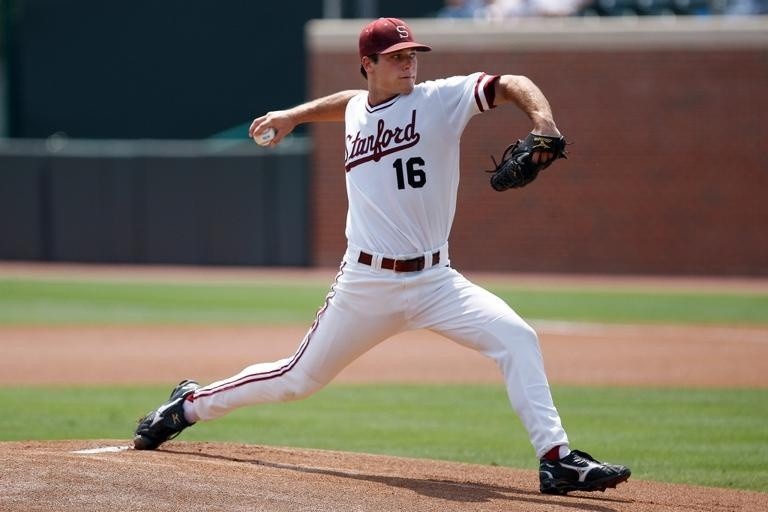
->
[358,17,433,62]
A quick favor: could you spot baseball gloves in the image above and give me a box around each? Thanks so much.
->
[490,133,565,192]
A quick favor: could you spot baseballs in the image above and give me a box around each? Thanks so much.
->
[250,126,274,146]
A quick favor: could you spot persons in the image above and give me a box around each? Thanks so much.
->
[131,14,632,494]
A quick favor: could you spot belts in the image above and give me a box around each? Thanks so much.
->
[358,250,440,273]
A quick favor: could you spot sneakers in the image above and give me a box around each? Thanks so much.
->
[539,449,632,494]
[133,378,202,449]
